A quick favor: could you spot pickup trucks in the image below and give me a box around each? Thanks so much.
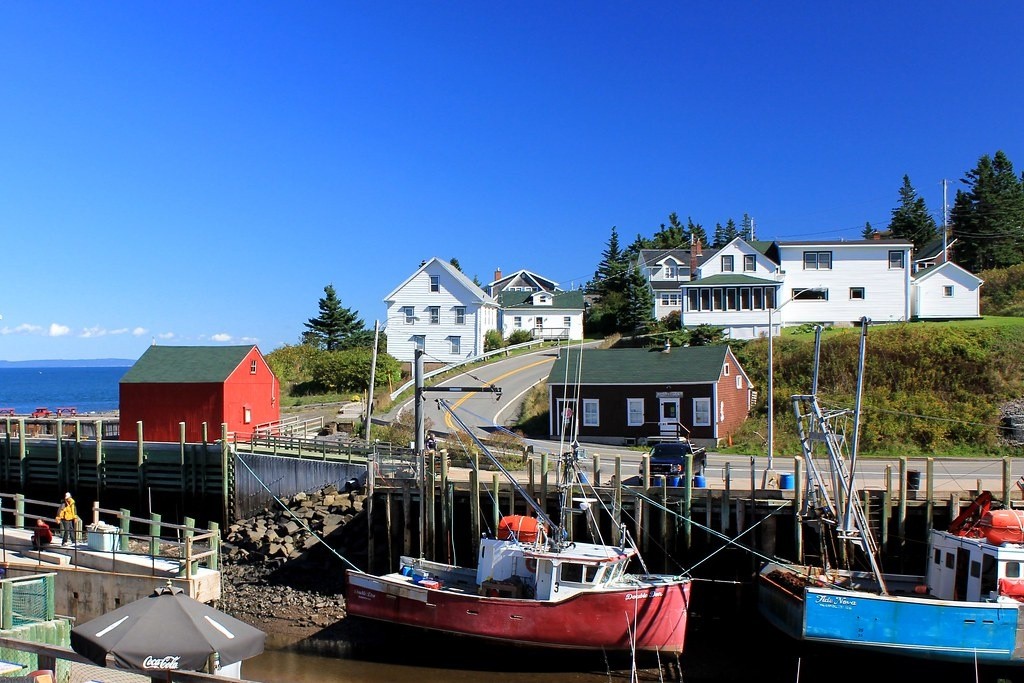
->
[639,443,707,485]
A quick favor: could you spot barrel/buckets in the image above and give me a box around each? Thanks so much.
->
[653,475,664,486]
[694,475,706,488]
[680,475,686,487]
[576,472,587,484]
[669,474,680,487]
[779,473,794,490]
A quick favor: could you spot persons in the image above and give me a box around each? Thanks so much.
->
[31,492,78,552]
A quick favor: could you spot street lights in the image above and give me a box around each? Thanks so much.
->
[768,284,827,470]
[365,316,420,450]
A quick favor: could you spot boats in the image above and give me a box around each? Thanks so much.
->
[748,315,1024,660]
[346,322,692,657]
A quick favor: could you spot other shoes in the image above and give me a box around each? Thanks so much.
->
[63,542,68,547]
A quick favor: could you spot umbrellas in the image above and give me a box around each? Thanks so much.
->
[69,578,266,676]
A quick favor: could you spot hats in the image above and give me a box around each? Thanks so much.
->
[65,492,71,499]
